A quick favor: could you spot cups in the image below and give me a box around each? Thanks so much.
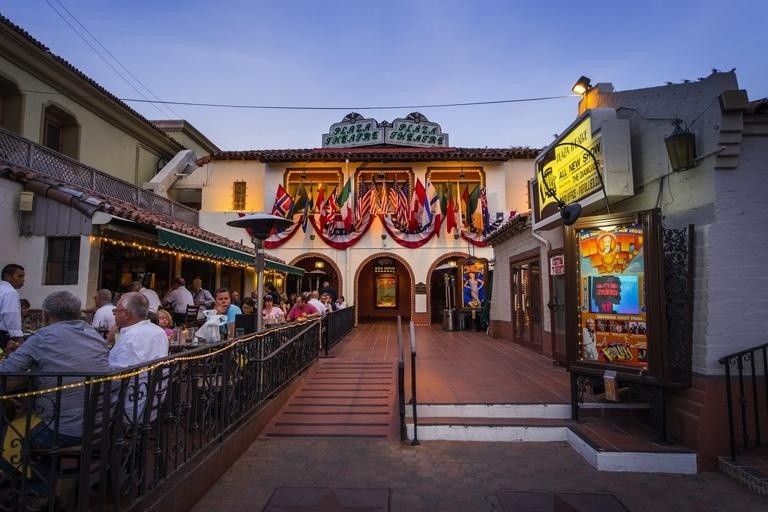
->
[266,314,286,323]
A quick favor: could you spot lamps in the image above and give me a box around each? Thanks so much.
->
[541,142,611,226]
[665,116,698,173]
[572,77,591,93]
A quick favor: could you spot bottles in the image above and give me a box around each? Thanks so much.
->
[178,325,199,346]
[204,310,228,343]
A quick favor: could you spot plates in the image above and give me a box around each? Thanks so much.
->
[23,332,31,336]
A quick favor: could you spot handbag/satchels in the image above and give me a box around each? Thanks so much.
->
[194,309,227,344]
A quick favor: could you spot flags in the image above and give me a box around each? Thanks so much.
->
[264,173,490,250]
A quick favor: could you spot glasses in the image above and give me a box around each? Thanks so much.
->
[112,308,127,315]
[93,296,102,300]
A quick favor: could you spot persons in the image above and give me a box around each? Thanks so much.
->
[158,277,347,375]
[464,272,485,307]
[630,324,637,334]
[582,318,599,360]
[597,320,608,332]
[638,349,647,362]
[602,236,613,254]
[637,324,646,334]
[614,321,628,333]
[1,263,173,510]
